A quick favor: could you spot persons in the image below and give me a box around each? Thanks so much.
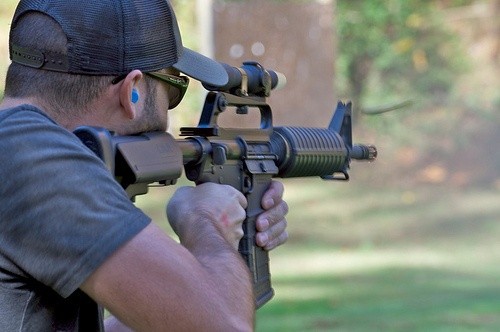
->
[0,0,289,332]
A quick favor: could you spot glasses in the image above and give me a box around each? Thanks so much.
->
[112,69,189,109]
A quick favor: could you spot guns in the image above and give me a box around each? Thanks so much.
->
[71,61,377,311]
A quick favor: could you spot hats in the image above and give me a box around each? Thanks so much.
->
[8,1,235,91]
